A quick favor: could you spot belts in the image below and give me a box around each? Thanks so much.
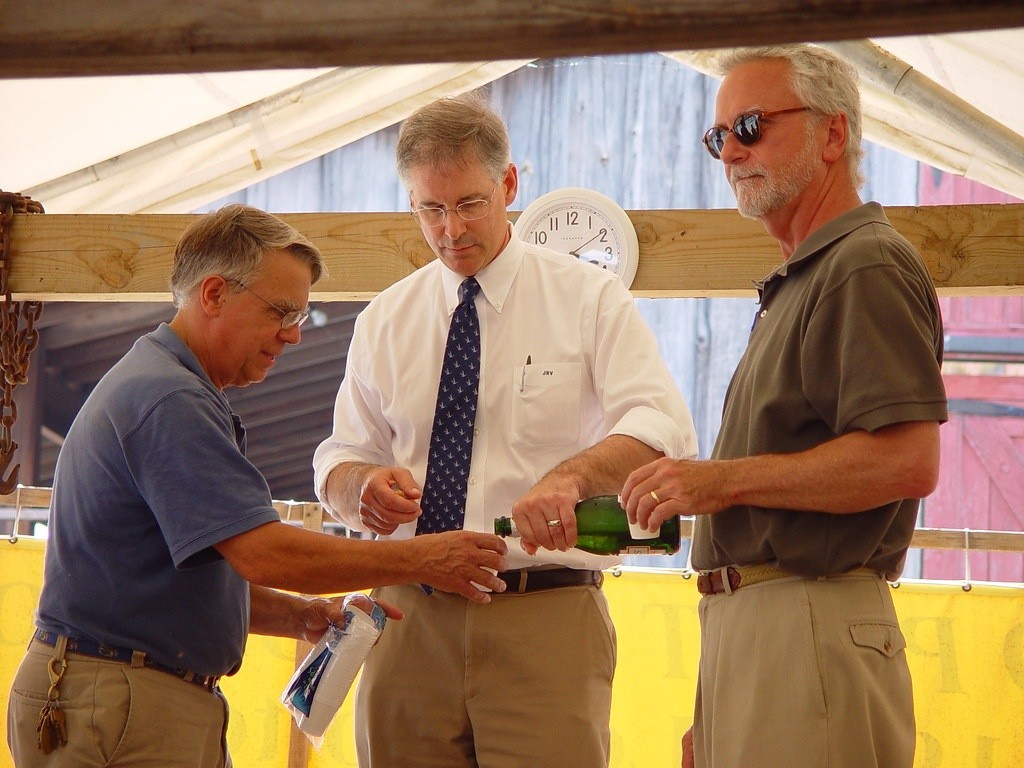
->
[695,564,884,596]
[492,568,601,593]
[36,628,220,692]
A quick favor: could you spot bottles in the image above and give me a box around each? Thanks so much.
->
[494,495,681,555]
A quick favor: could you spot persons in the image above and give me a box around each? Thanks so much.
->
[6,202,508,768]
[618,42,949,767]
[311,100,702,767]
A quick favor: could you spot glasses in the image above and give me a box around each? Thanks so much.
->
[408,171,499,229]
[223,274,310,330]
[701,106,812,160]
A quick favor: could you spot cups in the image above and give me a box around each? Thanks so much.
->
[470,549,499,592]
[289,605,377,736]
[627,512,660,539]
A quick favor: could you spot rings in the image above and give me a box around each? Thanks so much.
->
[546,519,562,525]
[651,491,661,505]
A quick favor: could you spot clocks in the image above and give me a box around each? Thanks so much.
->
[515,188,639,291]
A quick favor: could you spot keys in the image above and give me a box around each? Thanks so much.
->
[36,706,69,750]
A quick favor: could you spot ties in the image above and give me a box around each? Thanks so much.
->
[413,275,482,599]
